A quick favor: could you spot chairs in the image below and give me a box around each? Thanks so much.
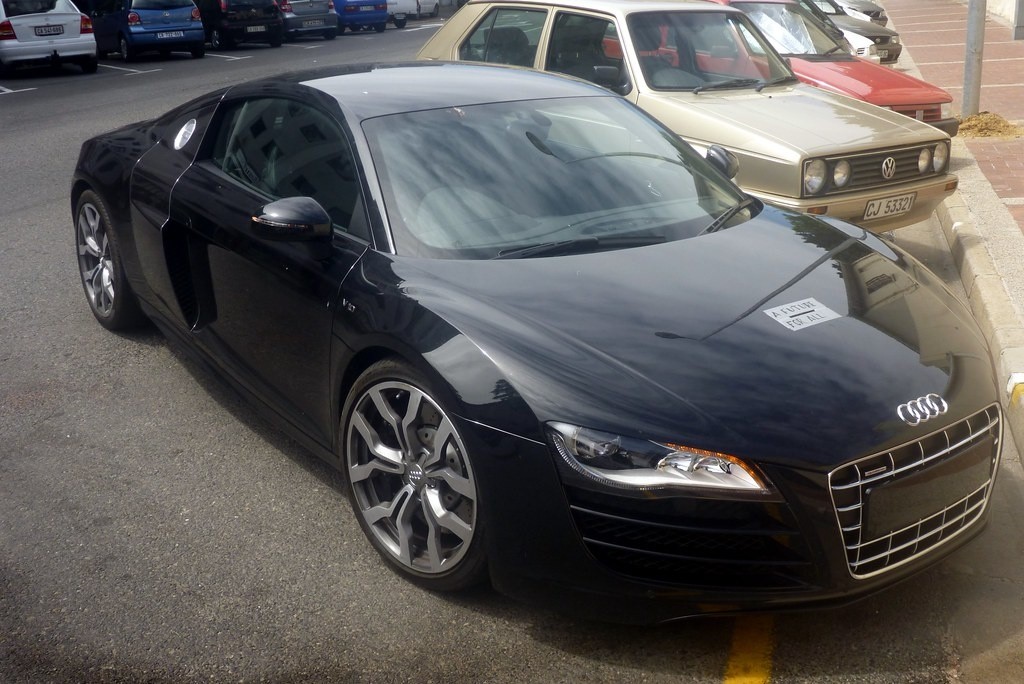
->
[484,18,672,90]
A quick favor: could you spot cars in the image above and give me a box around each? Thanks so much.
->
[276,0,337,41]
[835,27,880,65]
[333,0,389,35]
[413,1,957,232]
[602,1,957,136]
[835,0,888,26]
[0,0,98,76]
[795,1,903,65]
[88,0,206,63]
[196,0,284,51]
[70,63,1003,627]
[386,0,441,20]
